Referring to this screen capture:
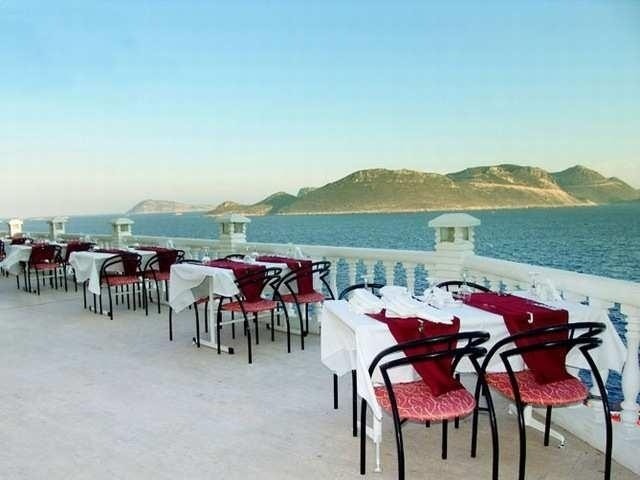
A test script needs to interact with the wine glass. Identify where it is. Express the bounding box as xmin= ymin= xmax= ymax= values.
xmin=202 ymin=247 xmax=211 ymax=266
xmin=361 ymin=274 xmax=375 ymax=296
xmin=457 ymin=271 xmax=472 ymax=303
xmin=243 ymin=246 xmax=253 ymax=266
xmin=528 ymin=271 xmax=540 ymax=305
